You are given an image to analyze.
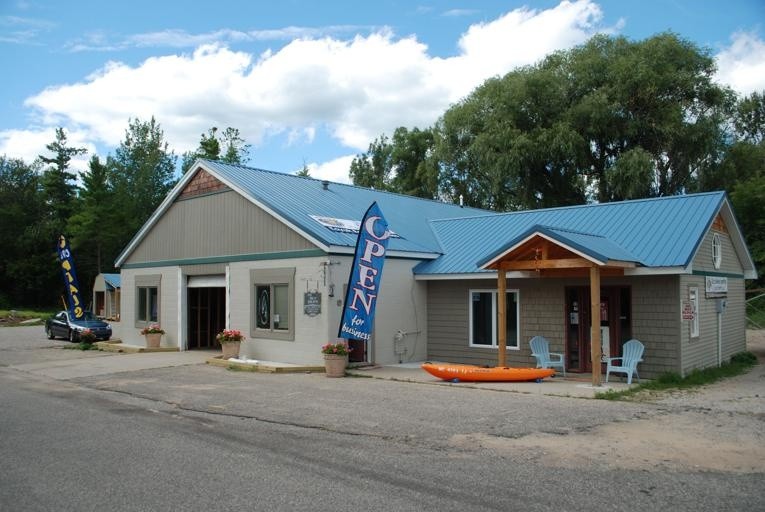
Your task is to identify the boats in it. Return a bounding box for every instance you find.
[419,360,558,384]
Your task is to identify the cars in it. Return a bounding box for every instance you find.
[44,310,113,343]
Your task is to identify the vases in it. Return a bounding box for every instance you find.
[145,333,161,349]
[82,338,93,345]
[324,353,347,378]
[221,341,240,359]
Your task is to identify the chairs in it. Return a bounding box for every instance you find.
[529,336,565,379]
[605,339,645,387]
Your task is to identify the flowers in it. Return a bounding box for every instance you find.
[79,328,96,339]
[321,337,352,355]
[216,329,245,343]
[140,323,166,335]
